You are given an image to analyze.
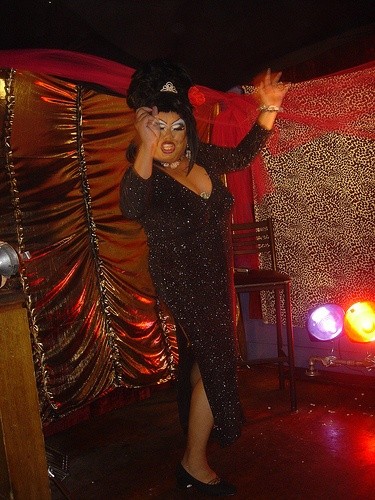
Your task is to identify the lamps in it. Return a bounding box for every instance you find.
[305,304,346,361]
[346,300,375,362]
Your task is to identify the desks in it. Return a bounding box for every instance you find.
[0,278,50,500]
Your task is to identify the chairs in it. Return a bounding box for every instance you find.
[231,219,295,411]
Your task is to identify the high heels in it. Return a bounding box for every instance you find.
[176,461,237,496]
